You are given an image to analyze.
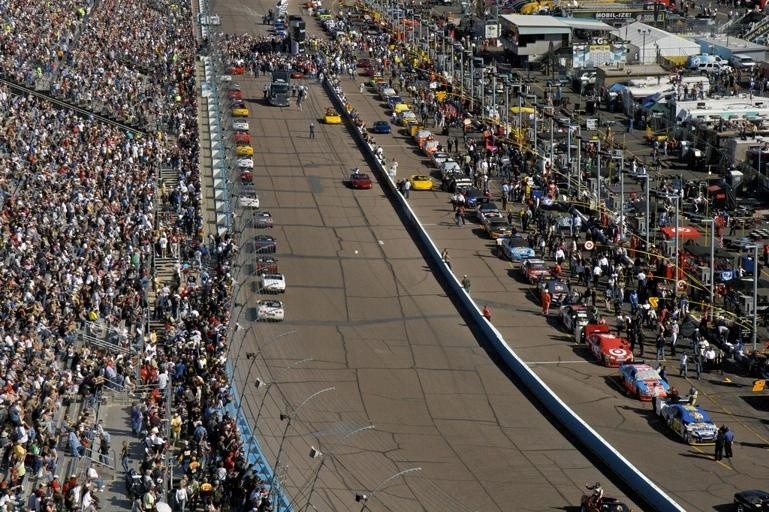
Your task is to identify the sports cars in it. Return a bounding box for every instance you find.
[581,495,633,511]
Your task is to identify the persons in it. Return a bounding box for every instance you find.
[0,1,768,510]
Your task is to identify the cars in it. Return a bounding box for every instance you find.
[219,66,289,322]
[733,490,769,511]
[267,19,288,40]
